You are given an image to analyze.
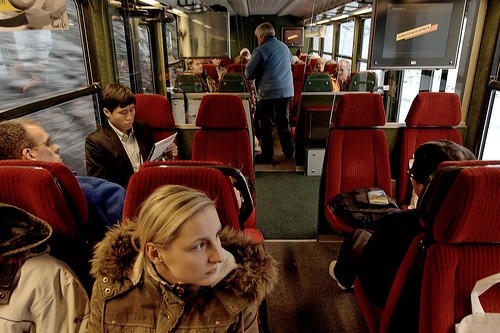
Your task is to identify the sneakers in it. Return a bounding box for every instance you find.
[329,259,355,291]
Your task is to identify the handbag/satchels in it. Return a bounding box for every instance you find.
[455,273,500,333]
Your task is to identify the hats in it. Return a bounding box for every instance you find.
[413,139,476,180]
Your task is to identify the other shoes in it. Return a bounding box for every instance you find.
[286,144,295,161]
[255,151,274,164]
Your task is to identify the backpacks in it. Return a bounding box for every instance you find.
[329,185,401,231]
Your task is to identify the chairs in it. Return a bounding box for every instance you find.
[122,160,256,251]
[0,159,94,289]
[130,94,179,161]
[351,161,499,331]
[323,94,394,234]
[418,165,499,333]
[399,92,463,228]
[191,93,256,206]
[172,53,381,140]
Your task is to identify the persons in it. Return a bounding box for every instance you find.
[245,22,295,163]
[290,47,358,92]
[0,120,126,238]
[329,140,479,309]
[315,26,325,37]
[85,83,178,187]
[212,47,259,104]
[186,56,220,94]
[0,200,91,333]
[84,184,282,333]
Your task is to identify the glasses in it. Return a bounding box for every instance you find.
[407,167,421,183]
[29,138,56,149]
